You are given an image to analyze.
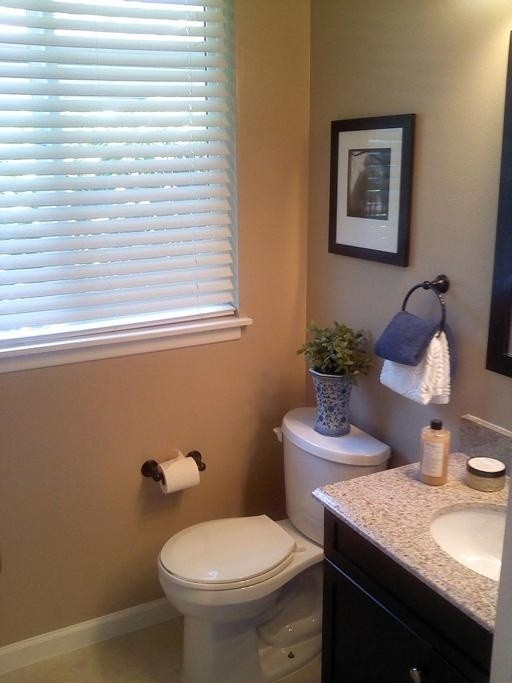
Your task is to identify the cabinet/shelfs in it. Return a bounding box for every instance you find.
[308,503,493,681]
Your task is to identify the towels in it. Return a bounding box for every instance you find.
[366,304,459,410]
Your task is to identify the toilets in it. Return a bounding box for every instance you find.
[156,404,392,683]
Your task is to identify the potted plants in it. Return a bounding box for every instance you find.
[296,317,376,439]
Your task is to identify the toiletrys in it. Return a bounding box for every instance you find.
[420,419,450,486]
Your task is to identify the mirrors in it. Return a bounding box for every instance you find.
[481,38,512,378]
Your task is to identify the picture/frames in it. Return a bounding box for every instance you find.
[327,110,420,269]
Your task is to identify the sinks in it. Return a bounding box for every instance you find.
[427,500,508,583]
